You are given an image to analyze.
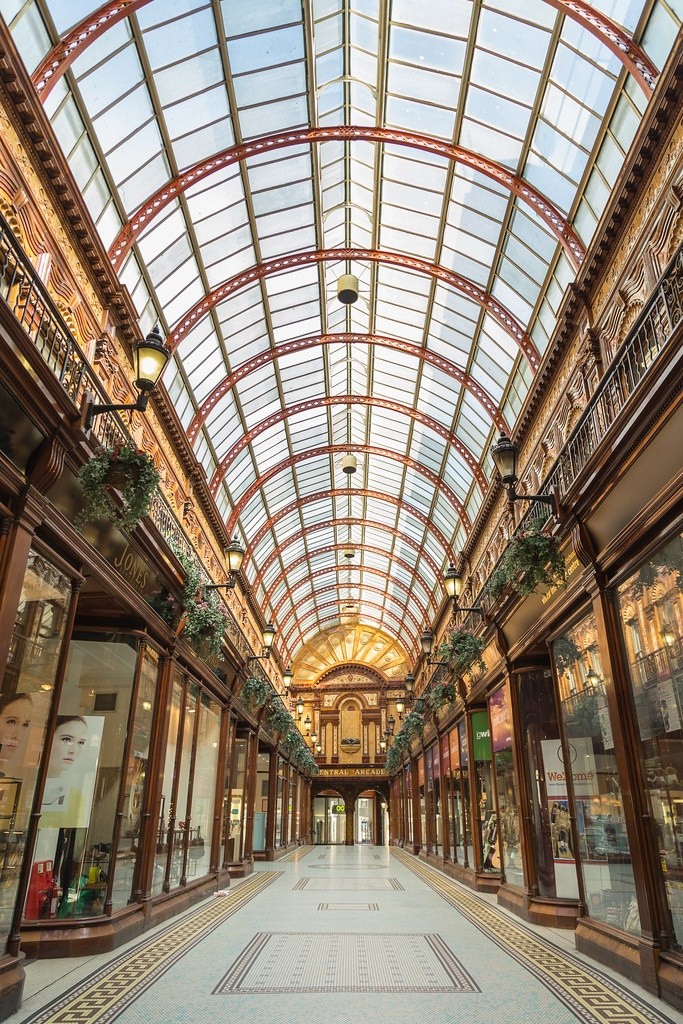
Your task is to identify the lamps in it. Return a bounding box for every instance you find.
[271,665,293,699]
[396,697,408,720]
[247,621,277,667]
[292,696,304,722]
[444,562,484,622]
[85,325,170,430]
[380,714,400,754]
[490,431,567,524]
[404,671,427,704]
[301,715,321,756]
[206,533,245,595]
[420,626,450,674]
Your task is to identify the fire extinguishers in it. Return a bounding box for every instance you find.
[39,879,61,919]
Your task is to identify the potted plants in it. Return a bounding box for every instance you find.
[340,738,361,754]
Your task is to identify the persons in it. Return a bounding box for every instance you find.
[559,838,568,854]
[0,694,35,829]
[38,715,94,828]
[600,827,627,851]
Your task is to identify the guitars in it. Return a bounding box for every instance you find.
[514,844,522,868]
[484,818,510,868]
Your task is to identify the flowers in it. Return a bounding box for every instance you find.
[430,681,456,706]
[185,596,230,663]
[76,442,158,531]
[435,625,487,675]
[487,518,569,597]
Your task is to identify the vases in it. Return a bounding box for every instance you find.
[201,624,217,637]
[108,464,136,492]
